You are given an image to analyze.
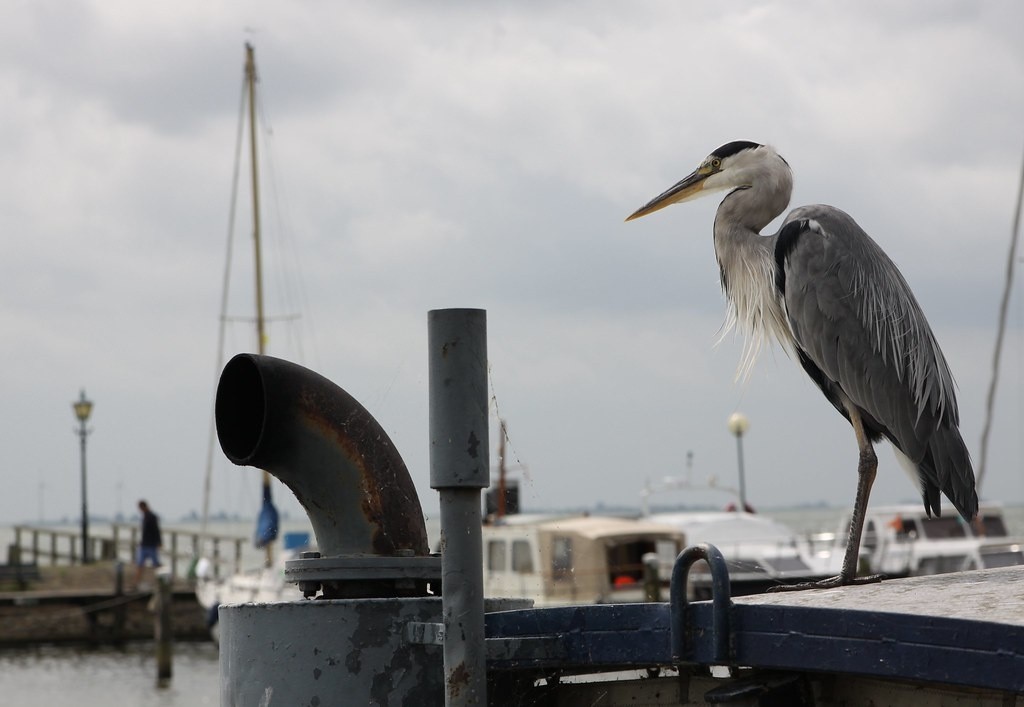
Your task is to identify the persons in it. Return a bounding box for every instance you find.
[138,501,162,566]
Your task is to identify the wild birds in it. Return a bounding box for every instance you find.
[623,138,982,591]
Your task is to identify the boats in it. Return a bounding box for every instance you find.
[192,39,1024,642]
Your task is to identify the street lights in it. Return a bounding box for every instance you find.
[65,388,96,564]
[728,413,746,513]
